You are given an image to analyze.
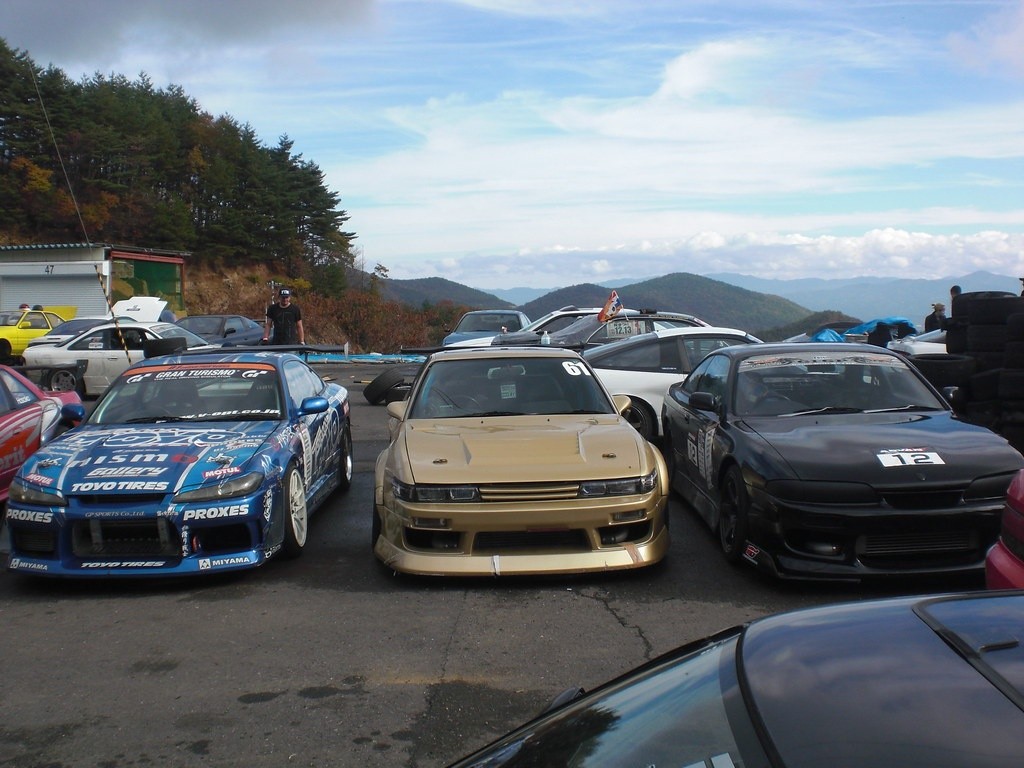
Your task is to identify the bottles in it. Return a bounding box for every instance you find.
[541,331,550,345]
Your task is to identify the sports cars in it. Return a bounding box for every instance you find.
[661,342,1024,592]
[578,327,878,441]
[4,343,353,579]
[0,364,83,507]
[373,342,671,577]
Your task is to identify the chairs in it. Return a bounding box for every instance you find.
[440,372,478,410]
[245,374,281,409]
[735,372,765,413]
[521,375,573,413]
[158,380,199,414]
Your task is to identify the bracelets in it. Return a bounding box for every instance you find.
[263,338,268,340]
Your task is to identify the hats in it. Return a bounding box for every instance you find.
[1019,277,1024,281]
[279,287,292,298]
[932,303,945,311]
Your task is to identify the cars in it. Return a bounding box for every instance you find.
[886,328,948,356]
[0,296,274,365]
[443,587,1024,768]
[442,304,712,354]
[20,321,245,402]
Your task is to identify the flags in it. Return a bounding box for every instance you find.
[598,290,623,322]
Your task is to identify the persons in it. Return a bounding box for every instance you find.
[924,303,946,332]
[260,288,305,346]
[19,303,29,308]
[898,322,916,339]
[33,305,43,311]
[869,322,892,347]
[950,285,961,298]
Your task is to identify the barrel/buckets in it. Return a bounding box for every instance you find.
[845,334,869,344]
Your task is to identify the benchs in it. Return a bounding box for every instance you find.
[200,394,247,411]
